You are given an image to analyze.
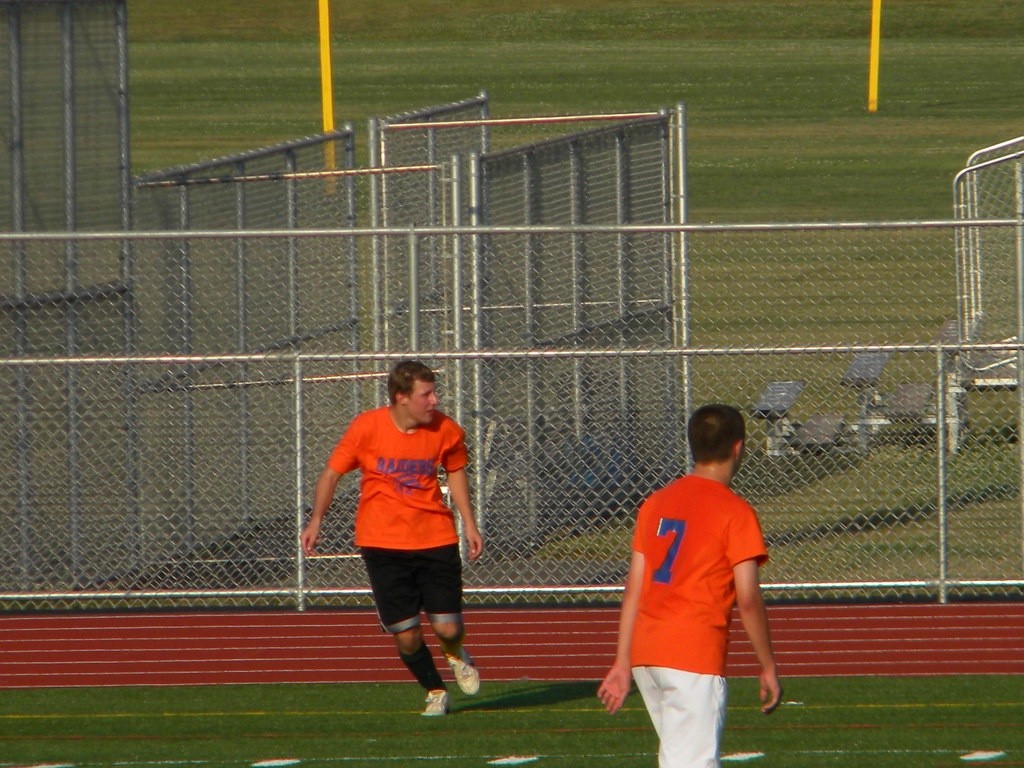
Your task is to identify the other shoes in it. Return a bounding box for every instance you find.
[440,644,480,695]
[421,690,448,717]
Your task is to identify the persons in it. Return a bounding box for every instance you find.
[299,357,484,715]
[598,403,783,768]
